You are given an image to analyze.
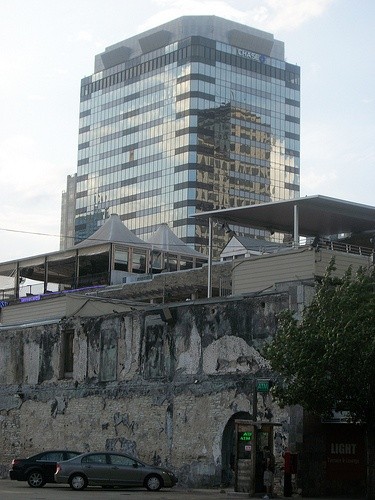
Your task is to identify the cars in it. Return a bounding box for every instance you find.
[8,450,83,488]
[54,451,177,492]
